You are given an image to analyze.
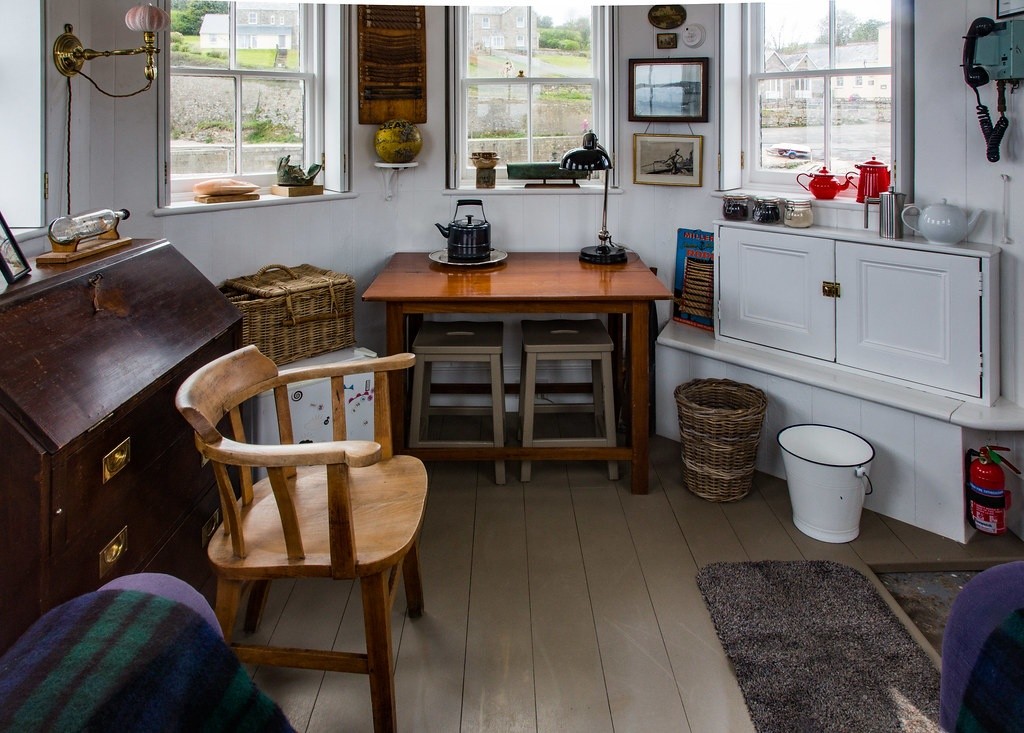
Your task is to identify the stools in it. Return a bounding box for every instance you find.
[408,320,507,485]
[521,318,621,482]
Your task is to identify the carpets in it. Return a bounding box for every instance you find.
[695,561,942,733]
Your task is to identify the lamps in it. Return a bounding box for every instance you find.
[559,133,628,265]
[54,1,171,101]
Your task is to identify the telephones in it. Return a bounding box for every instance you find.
[960,17,1024,87]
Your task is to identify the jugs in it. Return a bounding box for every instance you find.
[864,186,907,239]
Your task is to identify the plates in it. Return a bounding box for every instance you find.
[428,248,507,265]
[193,185,260,195]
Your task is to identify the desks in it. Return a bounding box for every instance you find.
[360,253,675,496]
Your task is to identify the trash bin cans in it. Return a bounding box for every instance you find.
[675,379,767,505]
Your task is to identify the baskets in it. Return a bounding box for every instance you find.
[673,378,766,502]
[215,263,355,366]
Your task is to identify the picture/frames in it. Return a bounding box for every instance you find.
[628,56,709,124]
[632,133,703,187]
[0,213,31,283]
[657,34,677,50]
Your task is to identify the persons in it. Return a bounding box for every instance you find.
[939,560,1024,733]
[0,572,297,733]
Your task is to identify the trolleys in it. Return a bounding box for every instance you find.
[766,143,811,159]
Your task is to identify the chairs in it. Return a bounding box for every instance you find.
[176,344,429,731]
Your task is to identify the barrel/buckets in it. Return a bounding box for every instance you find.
[777,424,875,543]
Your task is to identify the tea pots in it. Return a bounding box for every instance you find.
[901,198,986,246]
[846,157,892,203]
[435,199,495,263]
[796,167,853,199]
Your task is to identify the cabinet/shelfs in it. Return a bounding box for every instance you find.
[712,219,1002,407]
[1,238,243,657]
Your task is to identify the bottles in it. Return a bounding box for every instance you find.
[49,207,130,245]
[784,199,813,228]
[723,194,753,221]
[753,196,786,225]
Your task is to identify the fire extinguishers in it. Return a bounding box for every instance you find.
[968,445,1021,535]
